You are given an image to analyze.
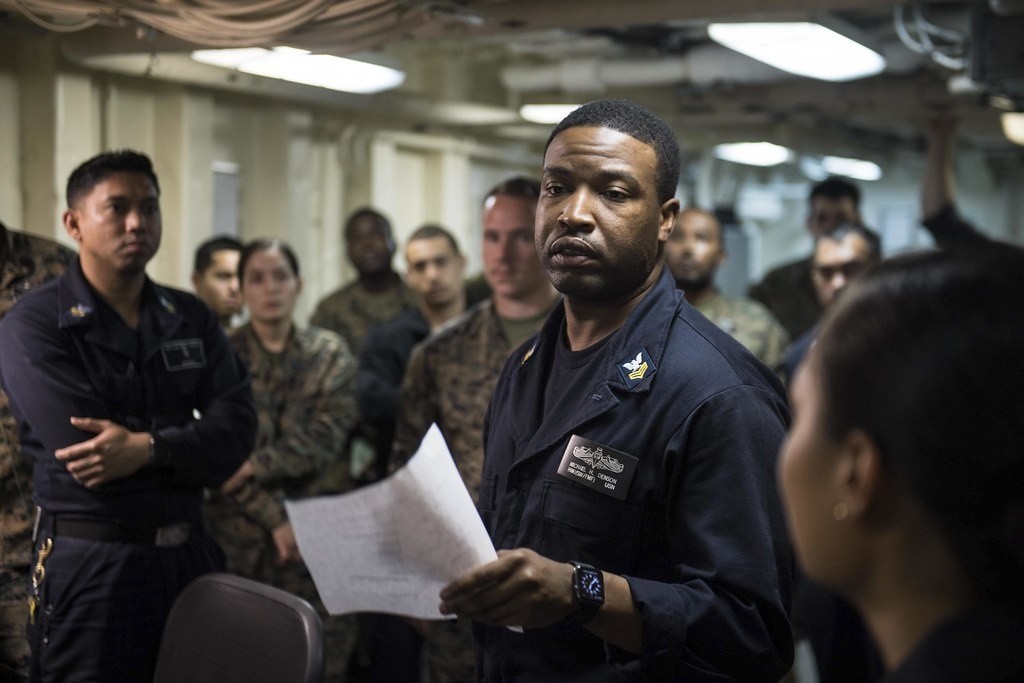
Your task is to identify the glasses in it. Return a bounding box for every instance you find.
[809,260,868,280]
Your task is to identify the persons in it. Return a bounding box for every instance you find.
[920,70,1024,277]
[386,177,569,683]
[750,175,886,375]
[775,240,1024,682]
[0,148,258,683]
[441,104,813,682]
[662,206,793,369]
[204,242,361,682]
[310,206,416,353]
[192,233,246,337]
[317,227,488,683]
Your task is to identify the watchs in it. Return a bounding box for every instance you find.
[557,561,606,628]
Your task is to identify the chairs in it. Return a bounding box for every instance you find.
[156,573,327,682]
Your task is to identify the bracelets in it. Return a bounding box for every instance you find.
[150,438,159,472]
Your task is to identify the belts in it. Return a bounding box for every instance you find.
[39,510,202,549]
